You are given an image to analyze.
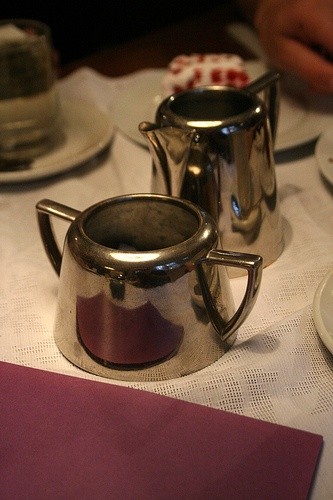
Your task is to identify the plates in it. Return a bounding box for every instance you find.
[0,93,114,181]
[310,264,332,362]
[112,63,327,153]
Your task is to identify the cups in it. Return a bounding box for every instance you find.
[138,70,286,276]
[0,19,66,162]
[35,192,263,382]
